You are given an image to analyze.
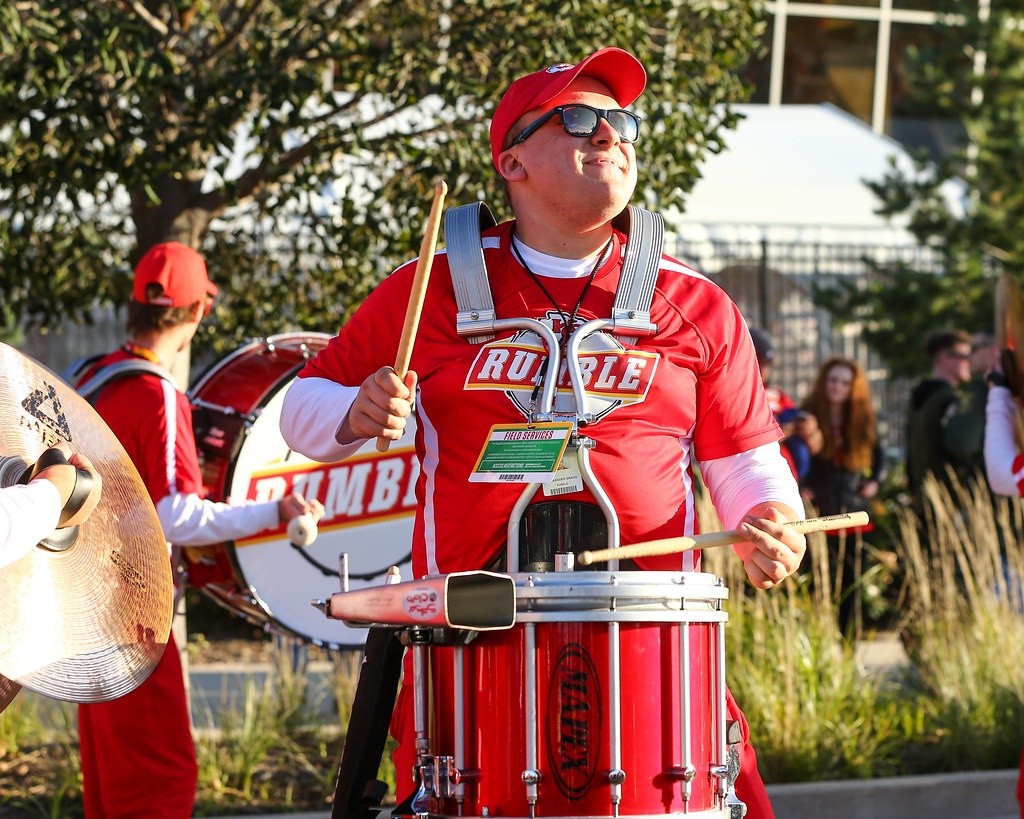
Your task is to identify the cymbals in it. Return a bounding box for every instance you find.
[0,339,177,708]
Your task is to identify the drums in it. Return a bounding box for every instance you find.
[185,327,422,651]
[395,566,735,819]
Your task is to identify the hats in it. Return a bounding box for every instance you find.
[133,243,218,306]
[489,48,647,176]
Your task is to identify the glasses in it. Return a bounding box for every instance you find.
[504,104,641,149]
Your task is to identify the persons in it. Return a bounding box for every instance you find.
[749,328,1023,633]
[280,43,804,819]
[0,243,306,819]
